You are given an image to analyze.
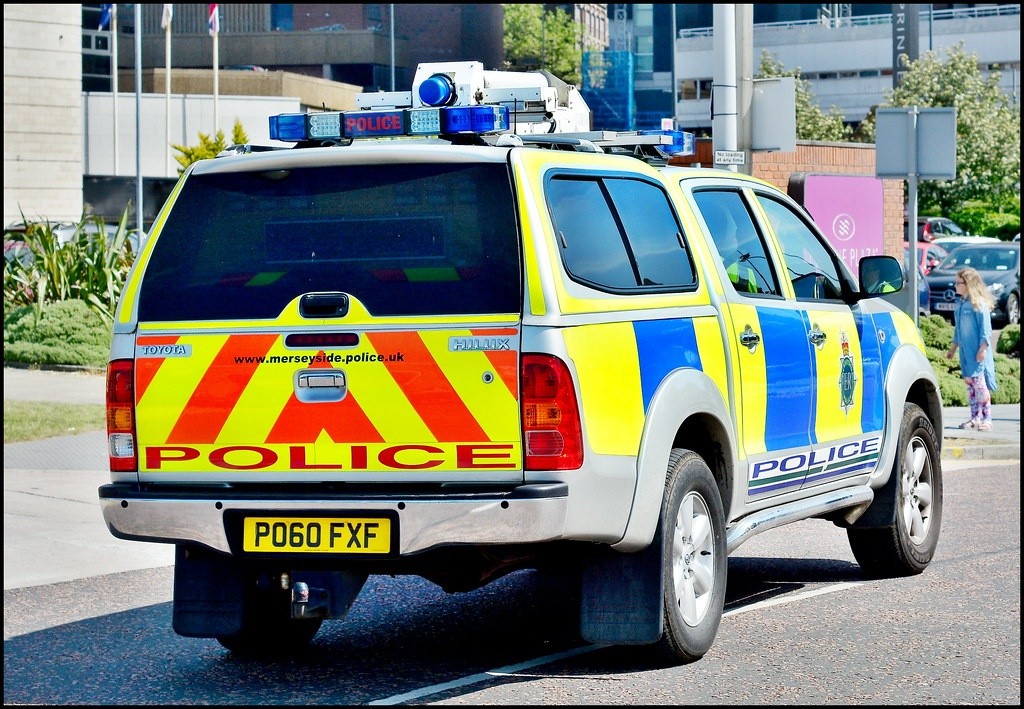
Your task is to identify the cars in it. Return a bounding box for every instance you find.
[4,219,144,301]
[903,216,1020,330]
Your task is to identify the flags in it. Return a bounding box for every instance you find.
[160,4,173,28]
[207,4,220,38]
[97,4,116,33]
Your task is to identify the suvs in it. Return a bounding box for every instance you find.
[98,59,944,663]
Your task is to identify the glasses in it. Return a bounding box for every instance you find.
[956,281,966,285]
[863,268,877,273]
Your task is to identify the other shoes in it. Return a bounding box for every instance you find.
[959,419,977,430]
[977,422,993,431]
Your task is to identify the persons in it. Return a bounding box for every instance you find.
[946,266,999,432]
